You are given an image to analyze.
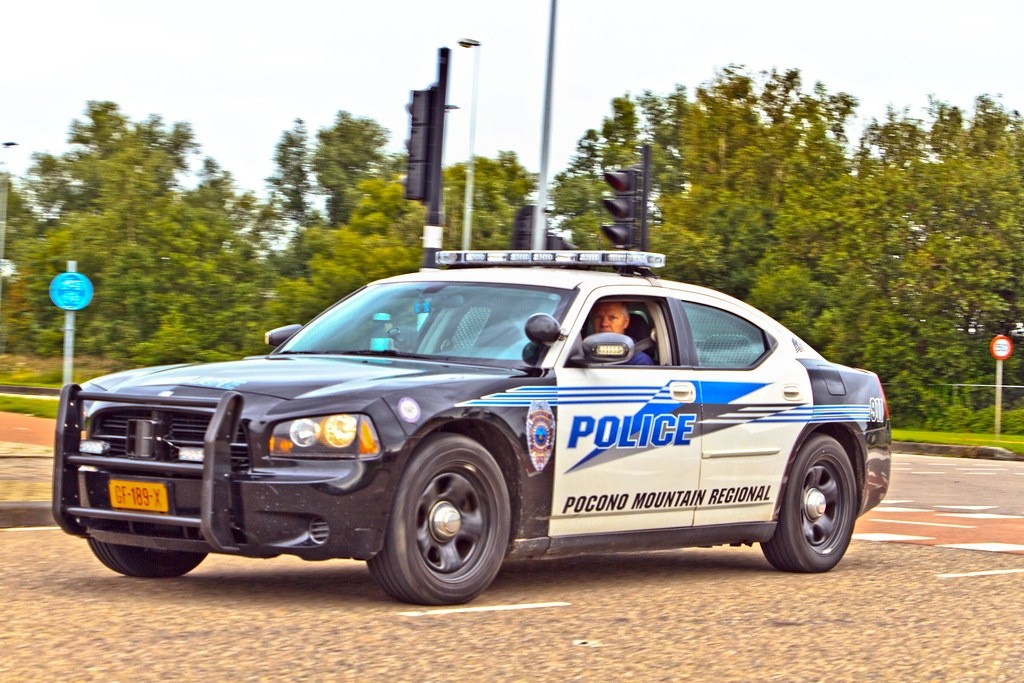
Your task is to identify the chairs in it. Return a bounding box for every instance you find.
[624,313,655,358]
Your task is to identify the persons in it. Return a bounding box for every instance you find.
[593,301,654,365]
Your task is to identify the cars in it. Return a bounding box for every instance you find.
[53,250,892,606]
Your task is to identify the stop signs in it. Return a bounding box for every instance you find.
[990,335,1013,359]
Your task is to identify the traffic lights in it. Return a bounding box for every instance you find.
[599,171,636,247]
[405,88,430,202]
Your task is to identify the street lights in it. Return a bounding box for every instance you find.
[458,37,481,249]
